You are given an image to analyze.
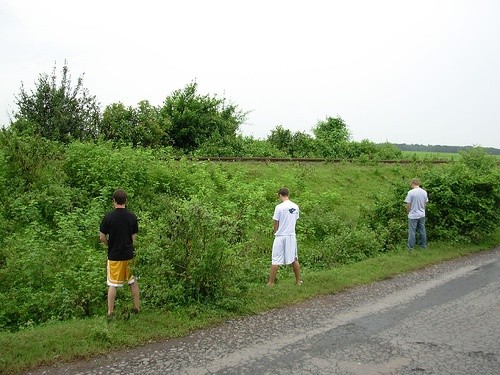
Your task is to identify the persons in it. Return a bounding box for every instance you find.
[404,178,429,249]
[99,189,141,317]
[264,188,304,287]
